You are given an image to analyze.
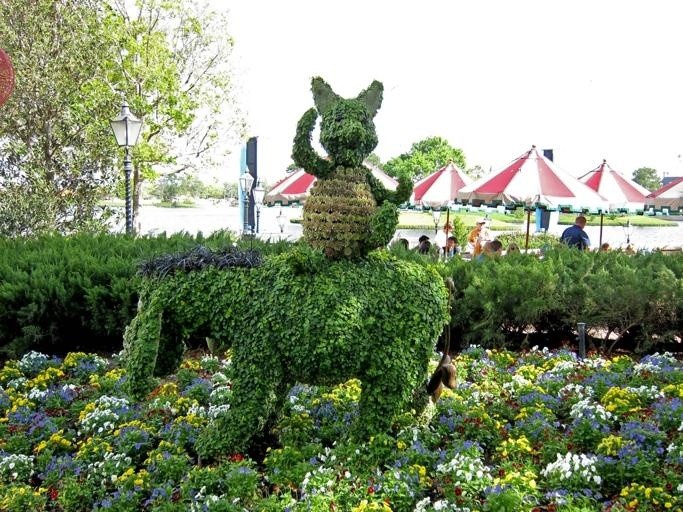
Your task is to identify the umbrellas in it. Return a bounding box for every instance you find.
[409,157,474,262]
[265,156,399,202]
[645,176,683,199]
[456,144,609,254]
[577,158,651,246]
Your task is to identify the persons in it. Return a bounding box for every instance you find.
[561,216,590,249]
[399,220,520,260]
[483,213,492,241]
[602,243,610,252]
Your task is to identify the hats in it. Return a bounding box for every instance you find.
[476,220,487,225]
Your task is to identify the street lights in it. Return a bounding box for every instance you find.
[621,219,635,249]
[276,209,287,233]
[106,97,146,235]
[239,166,252,236]
[252,179,264,233]
[430,208,442,234]
[482,214,492,232]
